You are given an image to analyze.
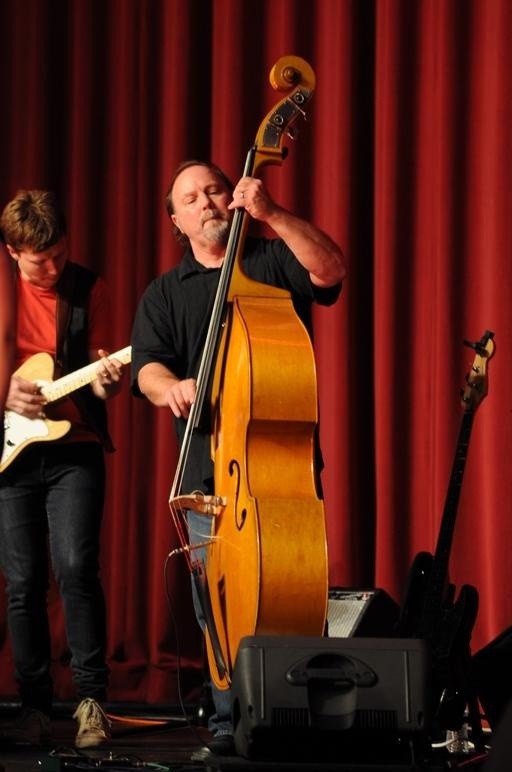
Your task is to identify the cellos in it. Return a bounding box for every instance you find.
[168,54,331,695]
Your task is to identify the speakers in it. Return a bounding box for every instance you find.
[326,587,402,638]
[232,635,426,753]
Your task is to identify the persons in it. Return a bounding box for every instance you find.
[1,189,124,750]
[127,159,350,763]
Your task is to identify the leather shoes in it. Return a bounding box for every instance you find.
[201,735,234,755]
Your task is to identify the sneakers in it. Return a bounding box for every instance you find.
[2,709,50,746]
[72,698,111,748]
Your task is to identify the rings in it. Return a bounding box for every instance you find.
[240,191,245,198]
[103,373,110,378]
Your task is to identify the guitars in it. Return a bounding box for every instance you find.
[0,345,133,474]
[409,330,496,665]
